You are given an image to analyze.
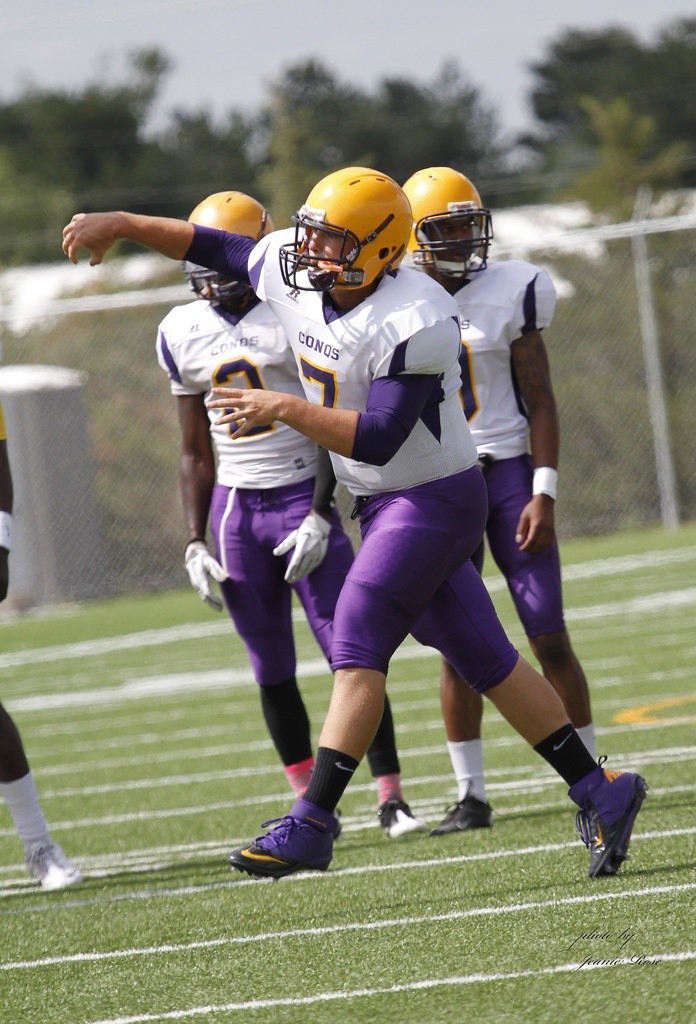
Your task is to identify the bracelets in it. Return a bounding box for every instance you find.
[185,538,207,553]
[532,467,558,501]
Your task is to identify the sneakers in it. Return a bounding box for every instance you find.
[568,755,648,878]
[429,781,493,835]
[227,799,339,880]
[27,844,81,891]
[378,797,424,839]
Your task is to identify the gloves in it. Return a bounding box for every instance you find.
[271,510,332,584]
[184,538,228,613]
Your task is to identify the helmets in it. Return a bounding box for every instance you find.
[278,166,414,292]
[401,166,495,279]
[181,191,274,308]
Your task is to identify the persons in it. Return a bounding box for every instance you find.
[63,166,649,879]
[1,392,81,892]
